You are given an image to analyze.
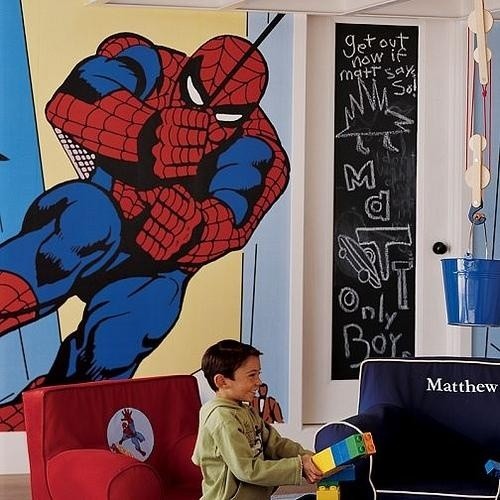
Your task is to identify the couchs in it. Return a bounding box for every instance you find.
[313,357,500,500]
[22,375,203,500]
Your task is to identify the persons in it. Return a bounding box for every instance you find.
[248,382,284,424]
[0,32,349,432]
[190,341,321,500]
